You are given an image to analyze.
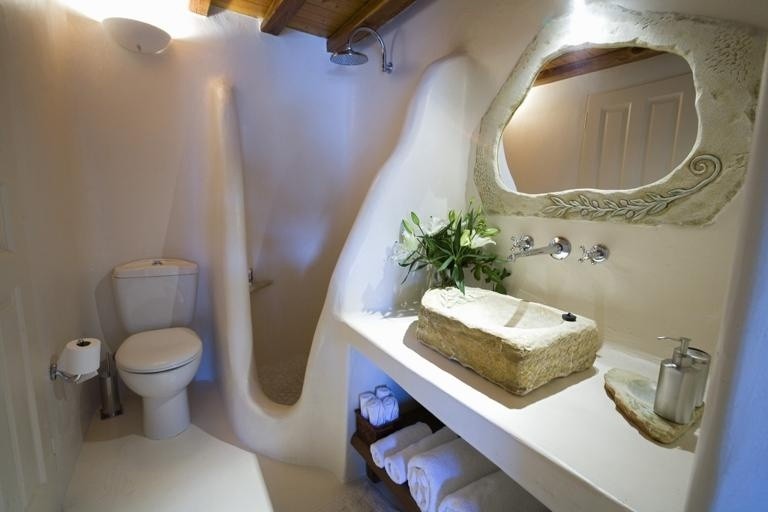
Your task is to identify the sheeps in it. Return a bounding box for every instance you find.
[509,237,571,261]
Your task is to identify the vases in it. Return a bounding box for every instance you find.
[392,199,511,295]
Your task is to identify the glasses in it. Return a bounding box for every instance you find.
[100,18,172,52]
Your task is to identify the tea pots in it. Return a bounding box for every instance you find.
[472,0,768,227]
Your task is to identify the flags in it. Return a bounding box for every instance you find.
[426,263,449,287]
